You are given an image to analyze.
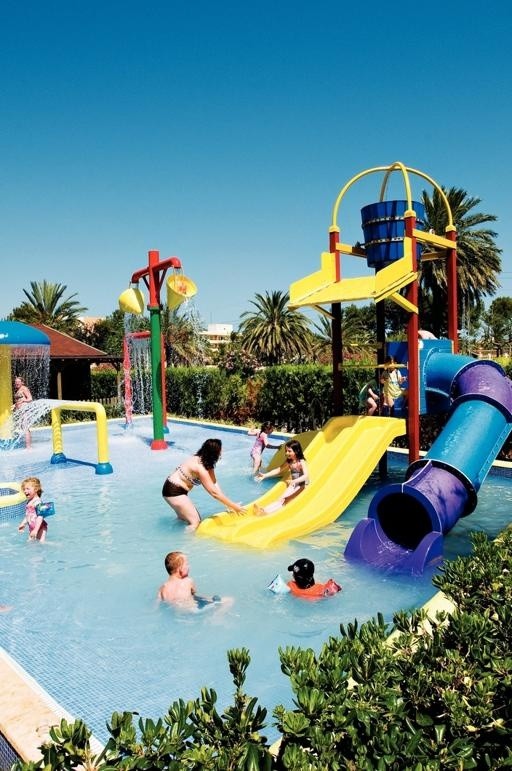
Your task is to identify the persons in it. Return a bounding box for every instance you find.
[162,438,249,534]
[157,551,234,625]
[251,420,284,478]
[18,477,47,545]
[380,357,407,416]
[253,440,309,515]
[286,558,342,601]
[358,370,388,415]
[13,376,34,449]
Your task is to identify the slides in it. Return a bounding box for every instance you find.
[345,338,512,576]
[196,416,407,548]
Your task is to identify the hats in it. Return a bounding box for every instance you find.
[288,559,315,577]
